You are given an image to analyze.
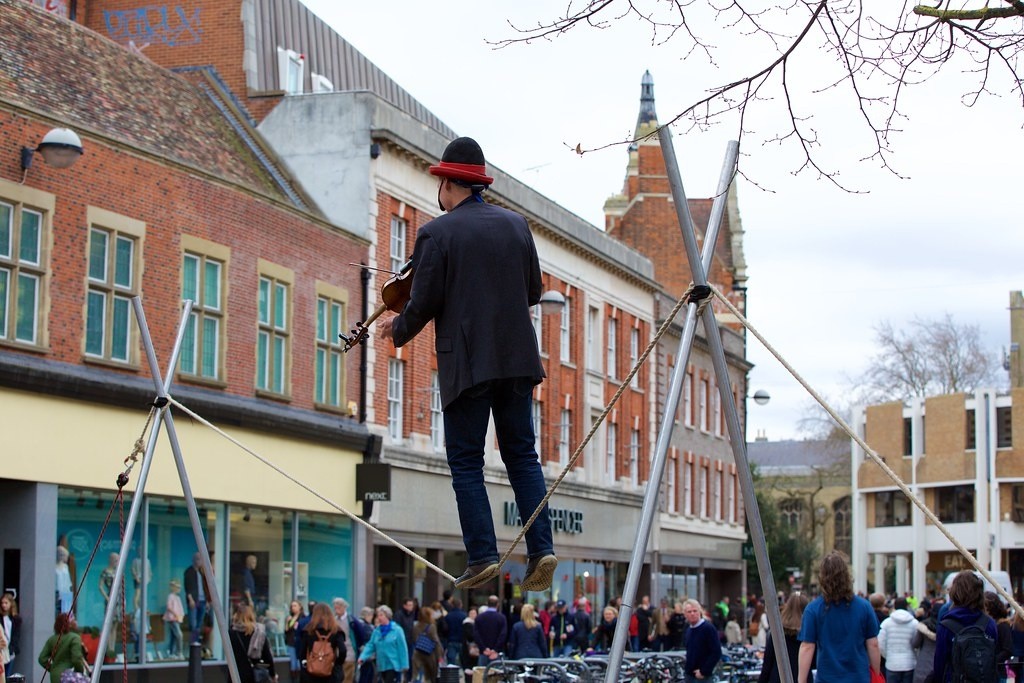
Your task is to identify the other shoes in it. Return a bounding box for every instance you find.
[455,559,499,589]
[519,554,557,592]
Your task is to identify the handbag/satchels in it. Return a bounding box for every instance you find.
[60,668,91,683]
[251,662,272,683]
[414,635,436,656]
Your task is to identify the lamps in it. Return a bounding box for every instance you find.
[22,126,83,169]
[94,490,104,509]
[263,509,272,523]
[75,488,85,507]
[539,290,566,315]
[745,390,770,404]
[242,506,250,522]
[165,498,173,514]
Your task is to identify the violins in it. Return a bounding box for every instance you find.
[338,264,414,353]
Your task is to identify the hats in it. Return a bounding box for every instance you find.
[556,600,566,608]
[428,137,493,186]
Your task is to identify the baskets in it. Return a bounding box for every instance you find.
[472,667,499,683]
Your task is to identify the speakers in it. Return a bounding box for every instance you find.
[355,463,392,502]
[370,143,386,156]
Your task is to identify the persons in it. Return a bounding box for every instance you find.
[0,552,1024,683]
[56,546,258,663]
[379,137,555,590]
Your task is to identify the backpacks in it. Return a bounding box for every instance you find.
[750,620,760,636]
[306,629,335,675]
[939,612,1000,683]
